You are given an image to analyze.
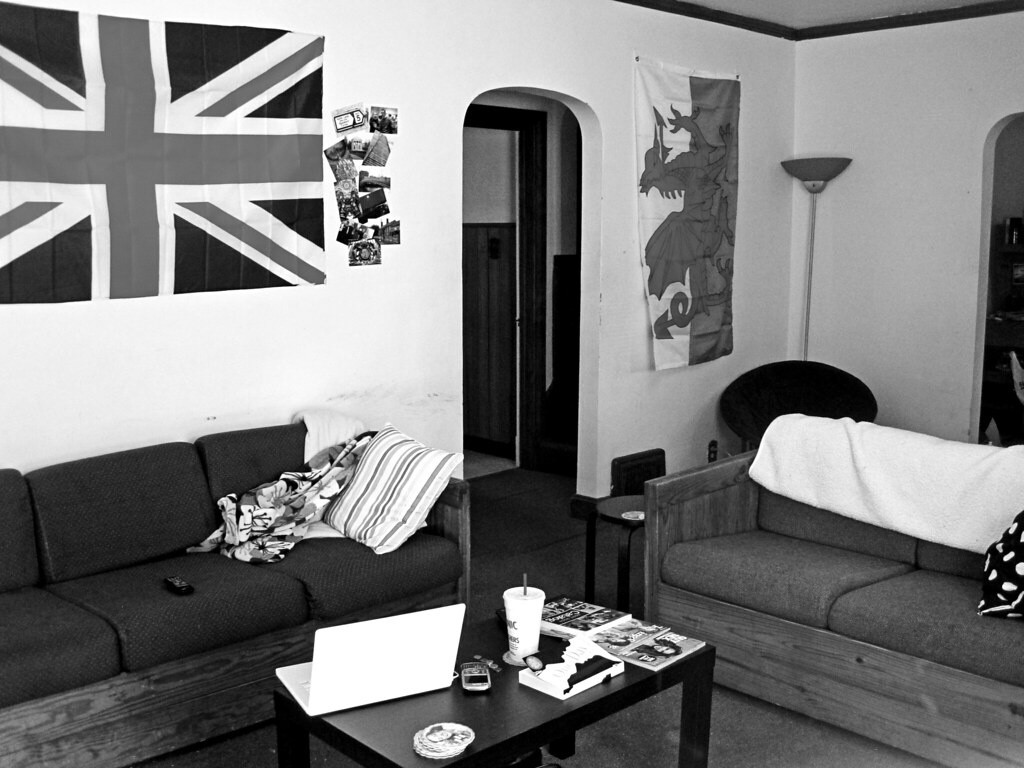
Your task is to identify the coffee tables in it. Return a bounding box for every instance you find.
[272,594,717,768]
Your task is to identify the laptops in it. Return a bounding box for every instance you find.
[274,603,466,717]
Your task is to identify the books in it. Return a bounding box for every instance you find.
[517,634,625,702]
[541,594,706,672]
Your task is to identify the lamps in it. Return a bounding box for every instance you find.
[780,157,853,359]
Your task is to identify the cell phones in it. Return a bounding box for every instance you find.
[461,662,491,691]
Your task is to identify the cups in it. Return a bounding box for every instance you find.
[502,586,546,662]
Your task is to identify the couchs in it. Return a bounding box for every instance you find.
[643,413,1024,768]
[0,422,471,768]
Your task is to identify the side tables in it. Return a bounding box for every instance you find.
[585,494,643,613]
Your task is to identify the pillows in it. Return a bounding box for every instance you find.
[976,509,1024,620]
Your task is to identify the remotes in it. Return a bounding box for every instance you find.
[165,575,194,595]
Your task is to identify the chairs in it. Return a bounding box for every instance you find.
[721,360,879,453]
